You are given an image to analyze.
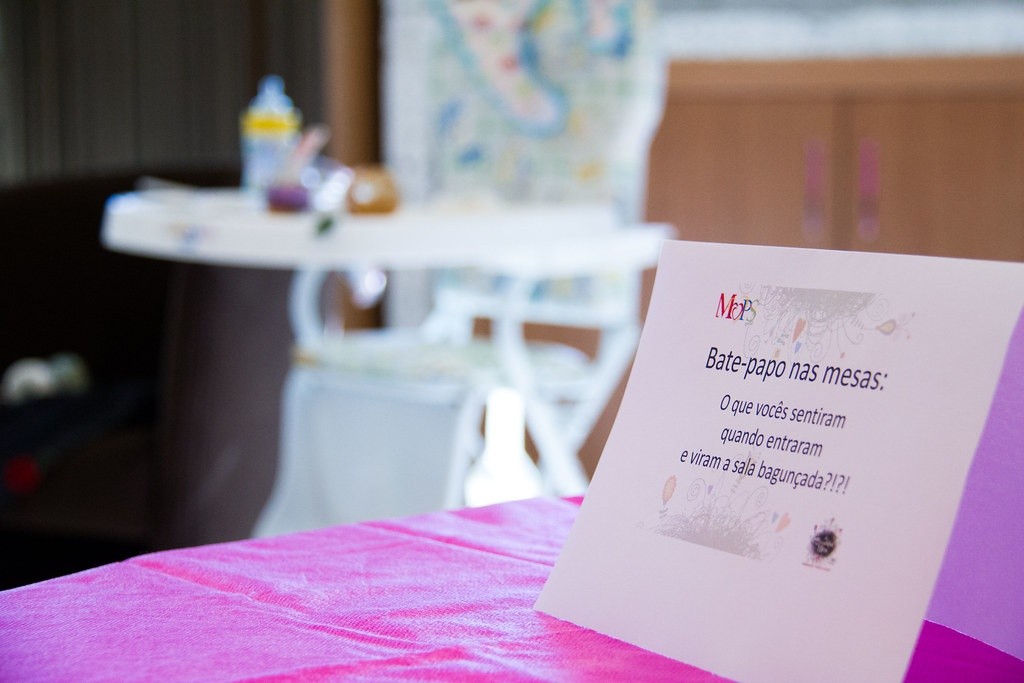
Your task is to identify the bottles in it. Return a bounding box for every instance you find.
[239,75,307,209]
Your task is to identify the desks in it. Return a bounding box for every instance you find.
[101,193,681,538]
[0,494,1024,683]
